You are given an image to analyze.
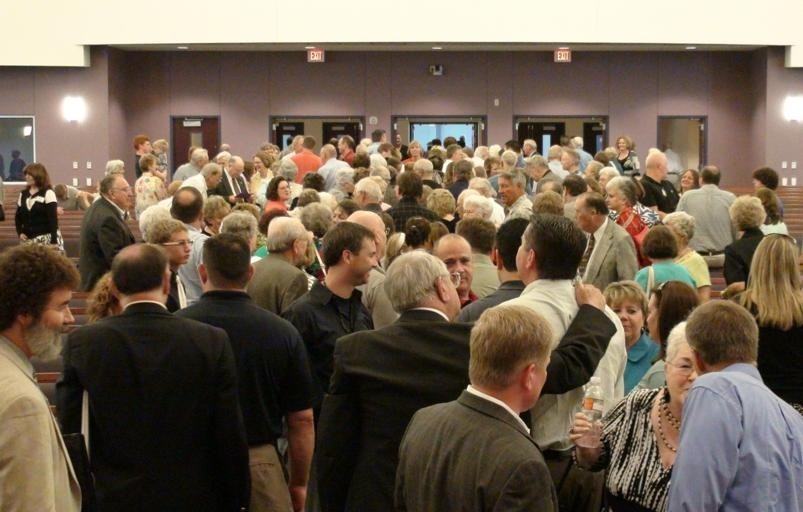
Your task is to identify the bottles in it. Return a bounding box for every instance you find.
[576,377,604,449]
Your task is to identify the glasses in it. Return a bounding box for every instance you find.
[449,273,461,287]
[161,240,193,246]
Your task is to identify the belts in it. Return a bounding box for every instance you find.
[698,251,724,255]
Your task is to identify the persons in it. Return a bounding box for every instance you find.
[55,242,252,512]
[1,244,84,510]
[394,306,554,511]
[666,298,803,510]
[5,149,26,180]
[57,130,802,511]
[14,164,65,252]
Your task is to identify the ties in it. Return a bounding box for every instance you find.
[580,234,595,277]
[175,275,187,310]
[233,178,240,195]
